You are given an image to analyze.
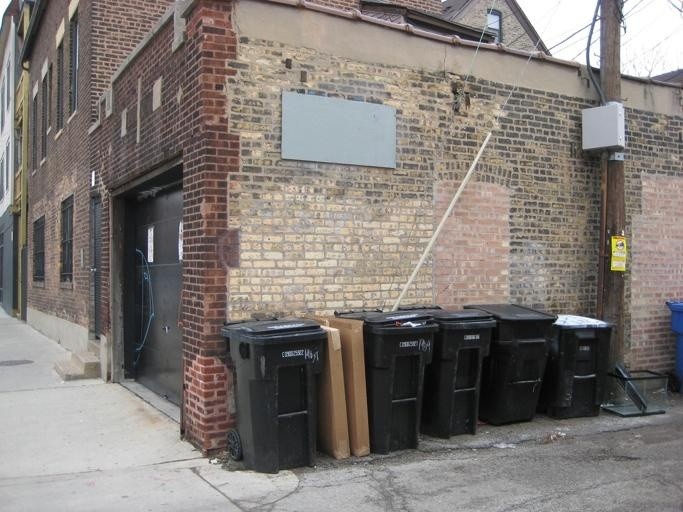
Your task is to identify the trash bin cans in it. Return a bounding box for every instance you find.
[335,308,440,457]
[399,305,497,439]
[665,301,683,394]
[220,317,330,475]
[464,304,558,425]
[536,316,613,418]
